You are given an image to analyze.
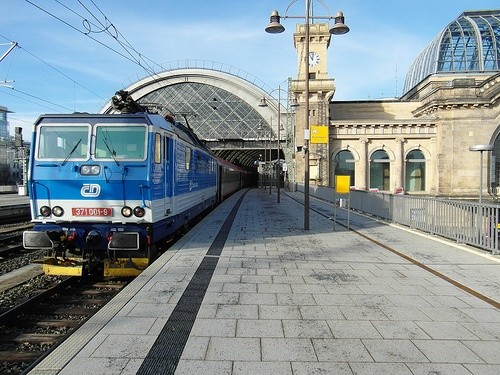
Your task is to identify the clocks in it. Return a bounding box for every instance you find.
[304,51,320,67]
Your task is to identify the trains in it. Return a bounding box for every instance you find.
[23,90,248,277]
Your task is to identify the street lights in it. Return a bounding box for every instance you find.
[259,84,300,205]
[258,122,284,196]
[265,0,350,230]
